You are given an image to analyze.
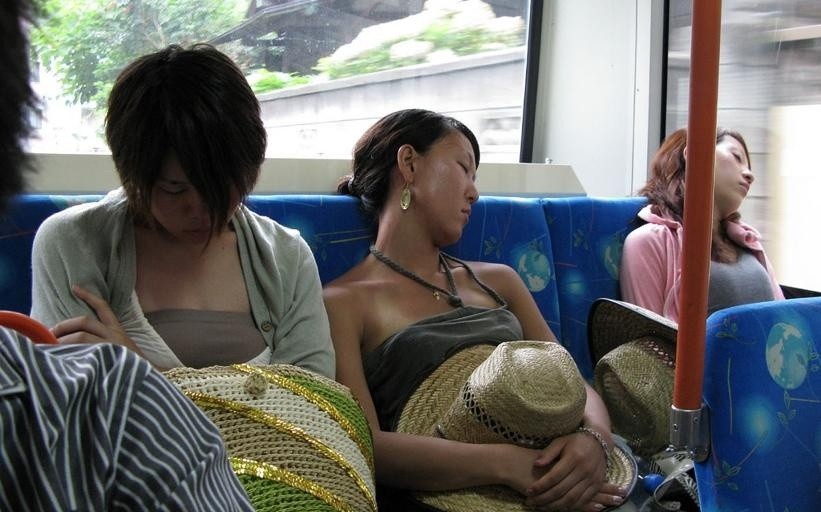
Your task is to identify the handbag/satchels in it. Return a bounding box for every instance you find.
[160,362,379,511]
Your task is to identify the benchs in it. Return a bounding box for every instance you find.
[1,187,651,512]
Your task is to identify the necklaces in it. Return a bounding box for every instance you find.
[392,255,443,303]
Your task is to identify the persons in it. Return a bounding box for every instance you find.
[1,1,257,512]
[322,106,637,509]
[619,124,789,511]
[29,41,337,380]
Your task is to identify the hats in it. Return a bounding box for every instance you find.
[393,336,642,512]
[584,294,678,463]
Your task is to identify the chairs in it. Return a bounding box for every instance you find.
[693,297,820,512]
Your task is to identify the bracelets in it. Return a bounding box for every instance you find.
[576,425,614,479]
